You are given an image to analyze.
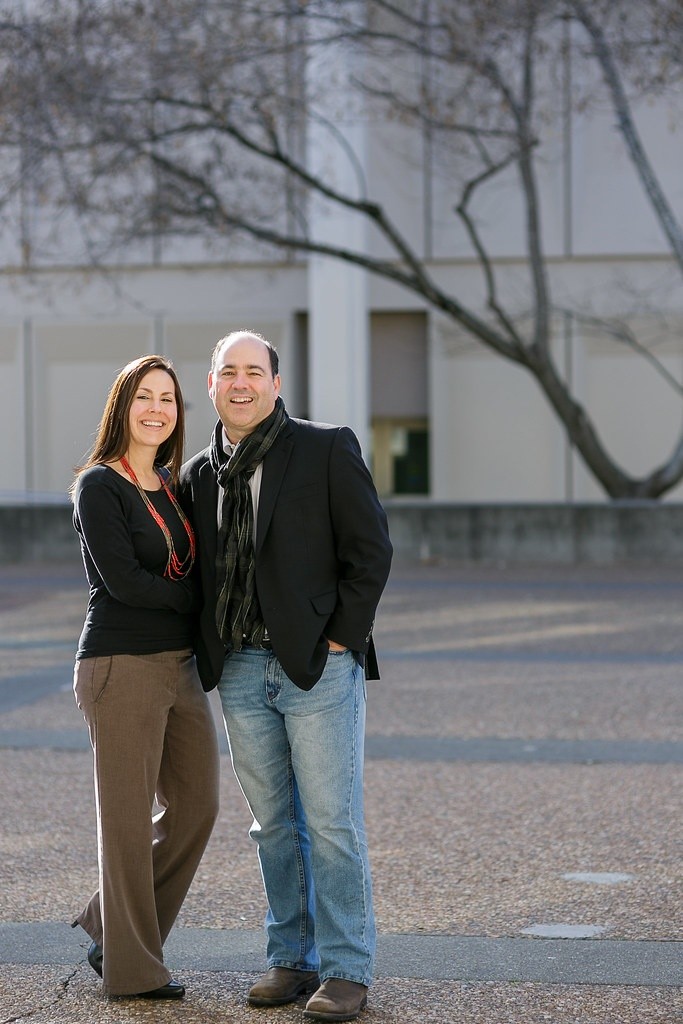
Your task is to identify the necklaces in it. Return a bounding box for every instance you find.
[120,453,197,580]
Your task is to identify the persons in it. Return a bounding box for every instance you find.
[180,329,392,1021]
[71,355,220,993]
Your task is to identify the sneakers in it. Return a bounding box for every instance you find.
[302,977,368,1021]
[246,966,320,1006]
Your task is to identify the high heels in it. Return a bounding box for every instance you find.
[71,919,103,978]
[134,979,185,999]
